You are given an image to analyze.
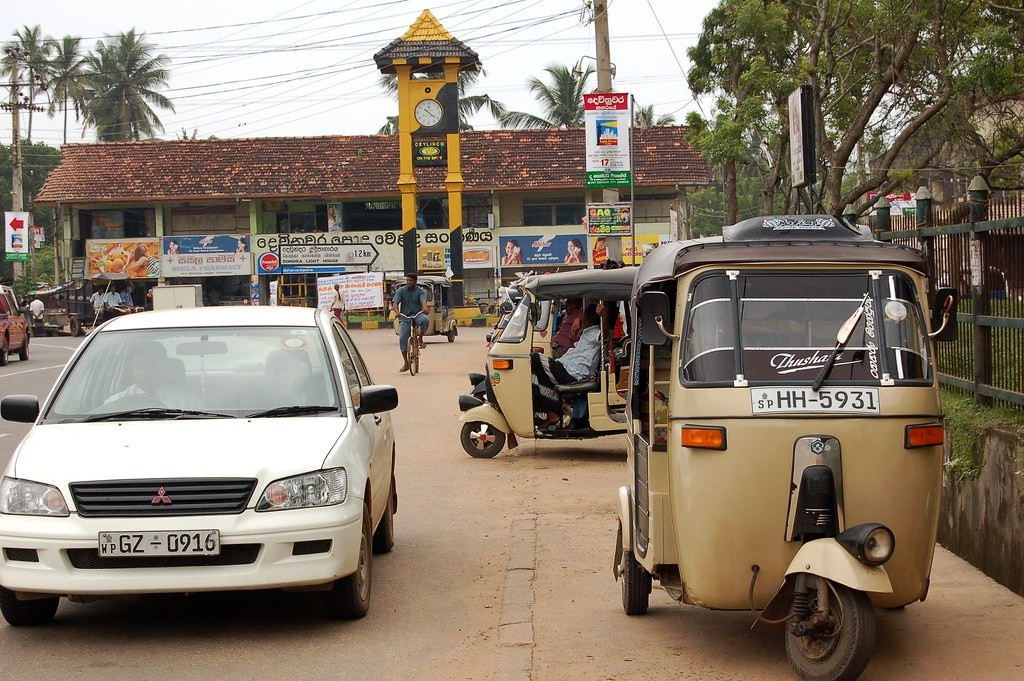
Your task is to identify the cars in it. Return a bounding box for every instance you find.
[1,306,399,622]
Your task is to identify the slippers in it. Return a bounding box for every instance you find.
[400,365,409,372]
[419,341,426,349]
[540,416,559,429]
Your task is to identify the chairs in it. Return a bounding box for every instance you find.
[161,358,186,393]
[240,351,319,407]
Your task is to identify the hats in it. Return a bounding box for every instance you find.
[128,341,167,369]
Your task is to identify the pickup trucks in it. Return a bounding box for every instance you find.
[0,284,31,366]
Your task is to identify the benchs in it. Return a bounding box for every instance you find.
[554,375,599,429]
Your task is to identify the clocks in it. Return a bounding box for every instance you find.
[416,100,443,126]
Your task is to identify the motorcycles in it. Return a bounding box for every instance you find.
[458,265,642,459]
[389,274,458,343]
[613,213,960,681]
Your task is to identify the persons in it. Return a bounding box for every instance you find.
[21,281,135,336]
[502,240,522,264]
[127,244,160,275]
[564,238,585,263]
[392,273,429,372]
[330,284,345,318]
[101,341,204,411]
[531,299,622,428]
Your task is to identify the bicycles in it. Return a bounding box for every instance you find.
[393,311,426,376]
[464,295,489,315]
